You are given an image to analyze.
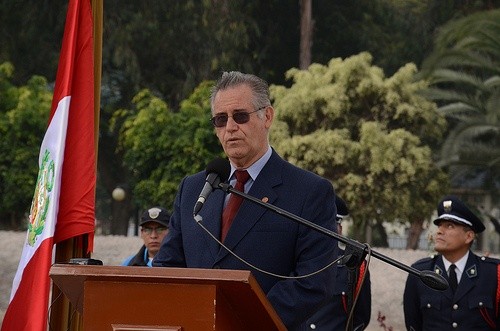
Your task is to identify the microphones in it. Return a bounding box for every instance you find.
[193,157,231,215]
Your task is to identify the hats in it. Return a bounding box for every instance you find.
[334,195,350,223]
[432,197,486,234]
[138,206,170,227]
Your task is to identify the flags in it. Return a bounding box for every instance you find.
[0,1,95,331]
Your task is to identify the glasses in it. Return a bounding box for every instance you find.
[209,106,267,128]
[142,226,167,234]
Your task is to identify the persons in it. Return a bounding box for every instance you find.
[335,195,372,331]
[149,71,340,331]
[121,207,171,268]
[403,196,500,331]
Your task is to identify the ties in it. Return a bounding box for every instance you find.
[218,169,250,244]
[449,264,458,295]
